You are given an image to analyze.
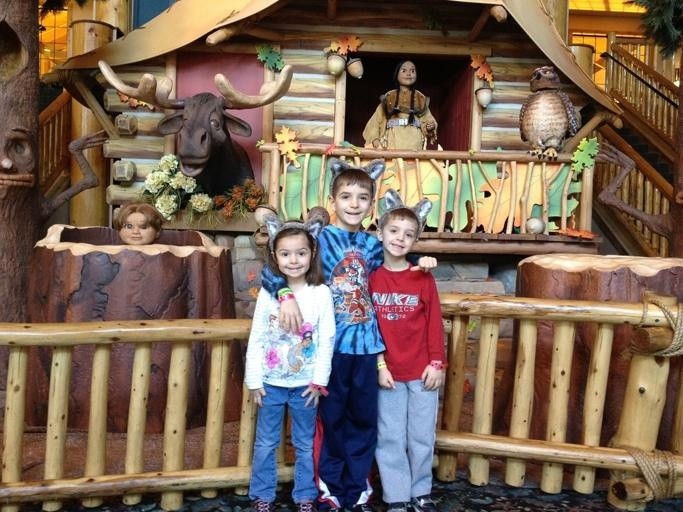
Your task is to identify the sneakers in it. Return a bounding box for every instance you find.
[318,496,435,512]
[249,499,271,512]
[295,500,317,512]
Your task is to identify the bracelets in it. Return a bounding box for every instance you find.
[428,360,449,370]
[276,288,291,297]
[277,293,296,302]
[375,361,388,369]
[307,382,330,397]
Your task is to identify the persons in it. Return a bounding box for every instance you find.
[375,187,447,511]
[359,58,442,152]
[112,203,166,246]
[254,159,438,512]
[241,215,337,512]
